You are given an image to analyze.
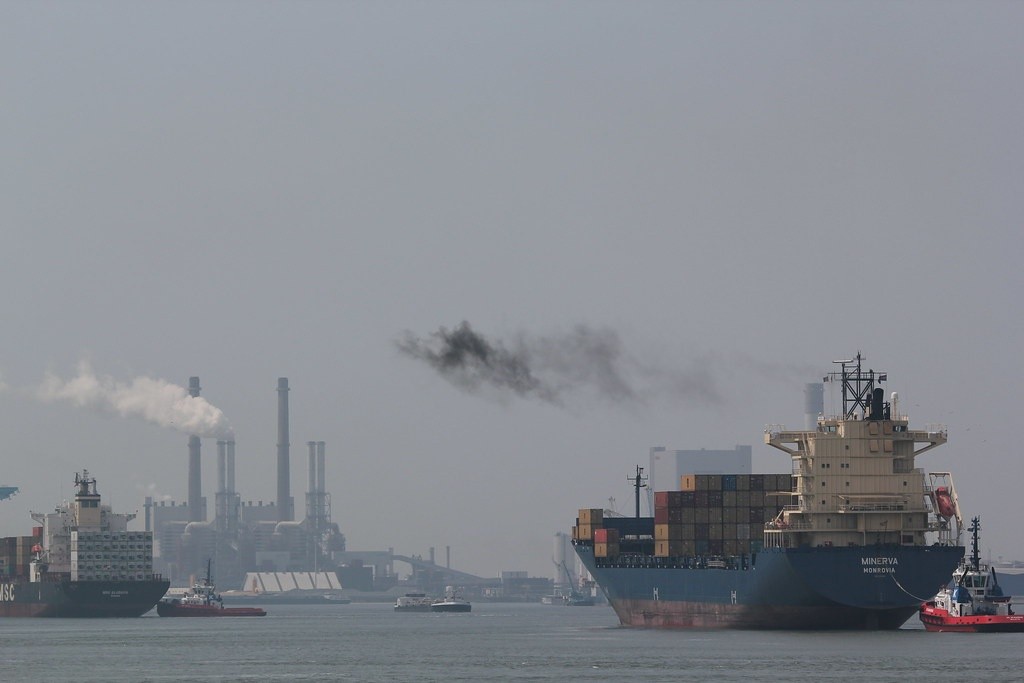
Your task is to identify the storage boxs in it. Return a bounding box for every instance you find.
[571,474,801,556]
[0,527,153,581]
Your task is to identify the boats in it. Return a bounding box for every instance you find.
[936,486,954,524]
[541,588,595,606]
[156,558,267,617]
[394,593,431,612]
[917,515,1024,632]
[570,348,968,630]
[431,585,471,612]
[0,469,171,619]
